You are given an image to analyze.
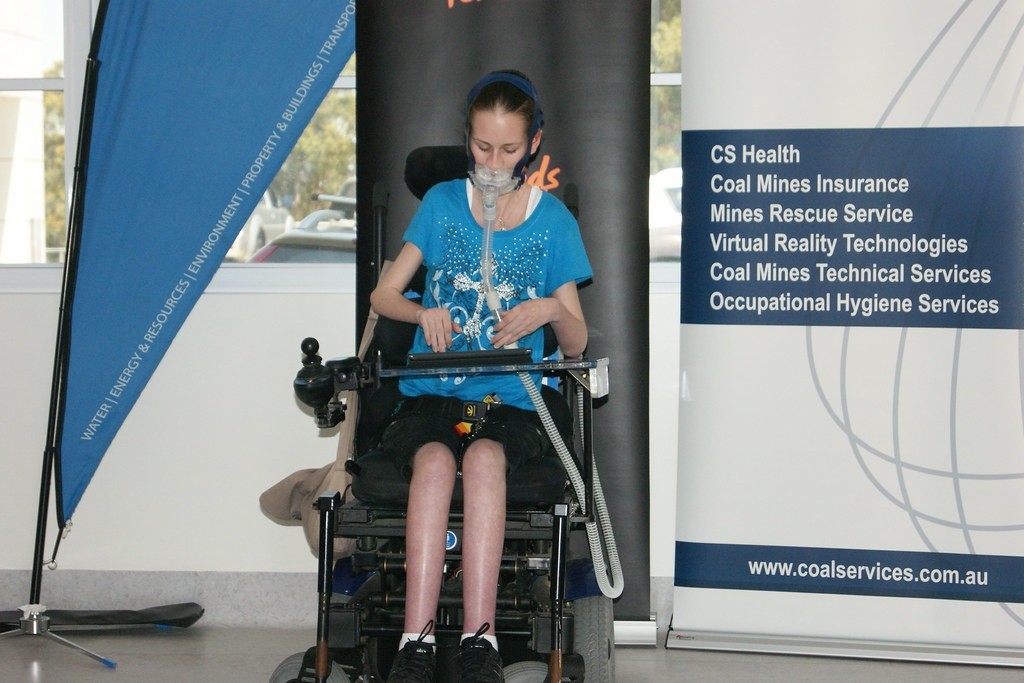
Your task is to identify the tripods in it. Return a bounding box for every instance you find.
[0,0,171,668]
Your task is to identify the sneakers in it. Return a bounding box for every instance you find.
[452,622,505,683]
[386,620,441,683]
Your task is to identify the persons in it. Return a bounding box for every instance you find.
[370,69,593,683]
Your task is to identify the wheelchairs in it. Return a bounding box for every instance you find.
[295,143,615,683]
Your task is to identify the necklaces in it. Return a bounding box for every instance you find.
[498,218,507,231]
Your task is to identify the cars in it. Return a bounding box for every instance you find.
[222,189,297,259]
[242,209,357,262]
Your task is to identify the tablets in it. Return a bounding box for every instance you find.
[406,349,532,367]
